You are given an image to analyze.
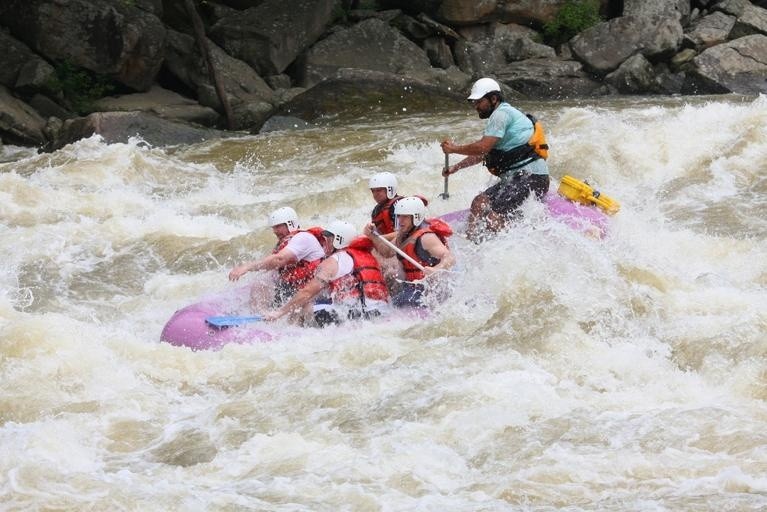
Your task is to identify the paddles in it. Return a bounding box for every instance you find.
[205,310,291,328]
[439,140,451,202]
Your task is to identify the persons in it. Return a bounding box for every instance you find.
[226,170,457,331]
[441,76,552,243]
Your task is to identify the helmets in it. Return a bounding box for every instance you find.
[268,207,299,233]
[368,172,399,200]
[325,221,354,250]
[393,196,425,226]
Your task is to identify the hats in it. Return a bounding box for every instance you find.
[466,77,501,100]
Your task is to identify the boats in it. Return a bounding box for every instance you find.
[158,185,612,354]
